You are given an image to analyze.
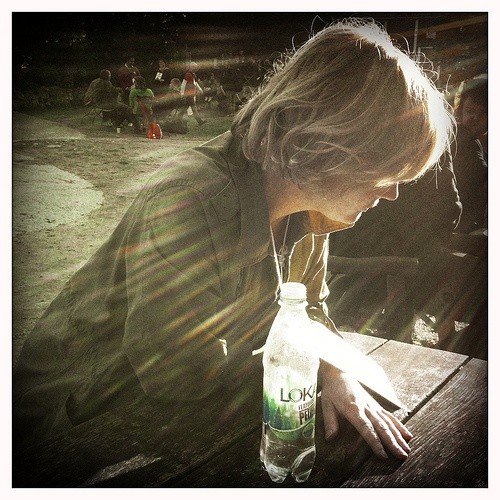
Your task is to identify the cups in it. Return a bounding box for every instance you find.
[117,128,120,133]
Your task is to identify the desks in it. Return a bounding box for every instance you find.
[12,332,489,488]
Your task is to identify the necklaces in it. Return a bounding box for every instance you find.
[277,214,290,278]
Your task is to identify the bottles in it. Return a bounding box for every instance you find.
[259,281,319,483]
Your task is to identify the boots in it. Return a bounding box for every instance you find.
[196,117,207,125]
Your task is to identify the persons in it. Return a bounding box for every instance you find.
[83,68,128,128]
[330,20,464,257]
[118,55,139,96]
[178,61,207,126]
[220,64,244,117]
[197,72,219,102]
[12,20,456,460]
[453,77,488,229]
[152,78,182,120]
[129,76,155,133]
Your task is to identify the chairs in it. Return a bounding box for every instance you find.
[80,93,112,126]
[326,255,419,343]
[132,95,154,129]
